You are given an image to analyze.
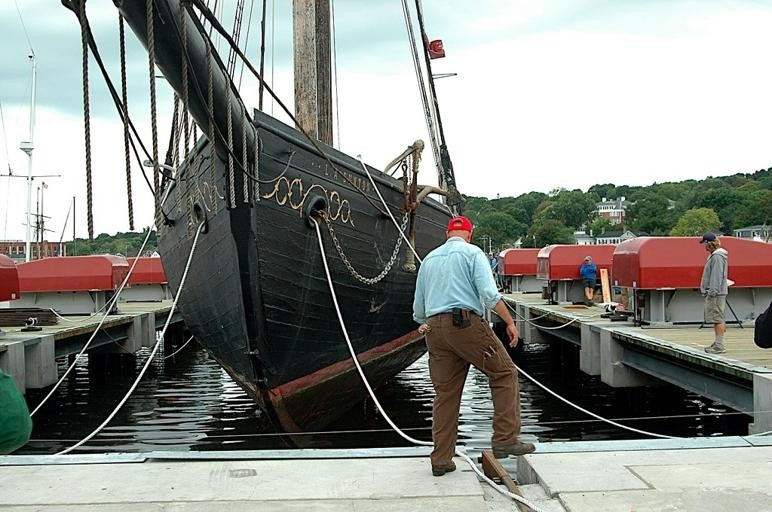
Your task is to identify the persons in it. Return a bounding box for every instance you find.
[579,255,598,301]
[492,246,502,288]
[698,232,729,354]
[505,245,512,249]
[412,216,536,476]
[487,253,497,279]
[485,252,489,260]
[0,369,33,456]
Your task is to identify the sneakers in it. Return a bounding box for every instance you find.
[706,343,727,353]
[432,461,456,476]
[493,439,536,459]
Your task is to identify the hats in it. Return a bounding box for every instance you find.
[700,231,717,243]
[585,256,591,261]
[447,216,473,232]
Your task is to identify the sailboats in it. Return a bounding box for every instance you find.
[60,1,461,449]
